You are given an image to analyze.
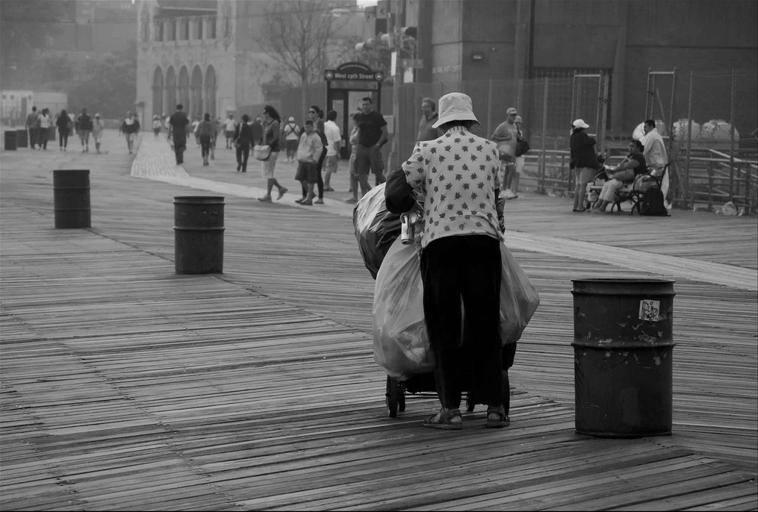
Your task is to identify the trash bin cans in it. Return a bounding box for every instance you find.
[5,131,17,151]
[53,169,92,230]
[49,126,56,141]
[17,130,28,147]
[173,195,225,275]
[569,276,677,437]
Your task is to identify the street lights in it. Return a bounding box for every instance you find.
[331,8,378,22]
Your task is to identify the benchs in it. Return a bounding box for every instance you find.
[585,162,672,215]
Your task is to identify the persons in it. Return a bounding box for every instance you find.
[75,107,92,153]
[589,137,608,180]
[569,119,589,211]
[346,113,372,205]
[294,121,323,206]
[323,110,343,192]
[346,96,388,204]
[26,106,40,149]
[513,115,522,195]
[223,113,237,150]
[152,112,161,136]
[92,112,104,155]
[416,97,443,143]
[490,107,519,199]
[46,108,51,118]
[192,117,200,132]
[257,105,288,202]
[585,140,646,214]
[642,119,672,210]
[384,93,511,432]
[283,116,300,163]
[308,105,328,205]
[55,109,73,151]
[121,110,140,155]
[194,113,214,167]
[168,104,189,165]
[234,114,254,173]
[37,108,51,151]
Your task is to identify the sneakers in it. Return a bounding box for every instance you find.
[314,200,323,204]
[592,209,603,214]
[498,191,511,200]
[586,207,592,213]
[257,193,272,202]
[294,199,303,203]
[507,189,518,200]
[311,192,316,199]
[300,200,312,205]
[276,188,288,201]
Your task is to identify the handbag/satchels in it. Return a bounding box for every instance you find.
[615,159,635,181]
[253,145,272,160]
[515,137,530,157]
[231,123,242,151]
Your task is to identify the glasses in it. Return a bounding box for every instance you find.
[308,110,316,114]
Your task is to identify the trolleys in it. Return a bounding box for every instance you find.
[384,198,511,417]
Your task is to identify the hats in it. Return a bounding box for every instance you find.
[573,119,589,129]
[432,93,480,129]
[506,108,517,115]
[288,117,294,122]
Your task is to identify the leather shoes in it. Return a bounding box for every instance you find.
[576,207,586,212]
[345,199,357,203]
[573,208,576,212]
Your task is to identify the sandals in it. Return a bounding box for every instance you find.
[423,408,462,429]
[485,406,510,427]
[325,186,334,191]
